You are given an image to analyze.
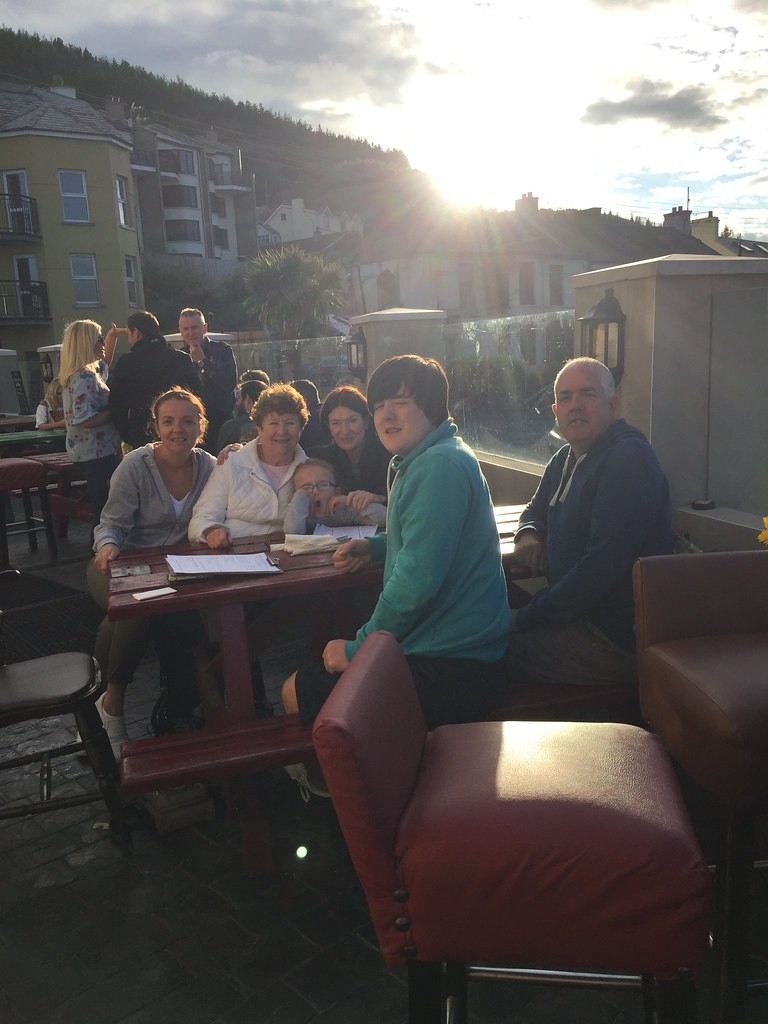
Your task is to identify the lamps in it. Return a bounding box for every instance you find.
[345,327,367,383]
[577,288,627,389]
[39,354,54,384]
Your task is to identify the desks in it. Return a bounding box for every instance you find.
[107,491,556,871]
[0,405,96,540]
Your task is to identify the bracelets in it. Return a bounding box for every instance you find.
[197,360,205,367]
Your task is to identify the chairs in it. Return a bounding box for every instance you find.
[633,548,768,904]
[309,629,714,1024]
[0,457,141,893]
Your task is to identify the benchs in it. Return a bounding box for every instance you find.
[121,709,343,871]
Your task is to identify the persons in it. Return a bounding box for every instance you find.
[281,356,511,803]
[35,308,392,760]
[496,359,672,687]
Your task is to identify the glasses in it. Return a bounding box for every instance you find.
[97,334,105,343]
[299,481,337,494]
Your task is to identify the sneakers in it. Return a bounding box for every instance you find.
[95,691,132,763]
[283,763,332,805]
